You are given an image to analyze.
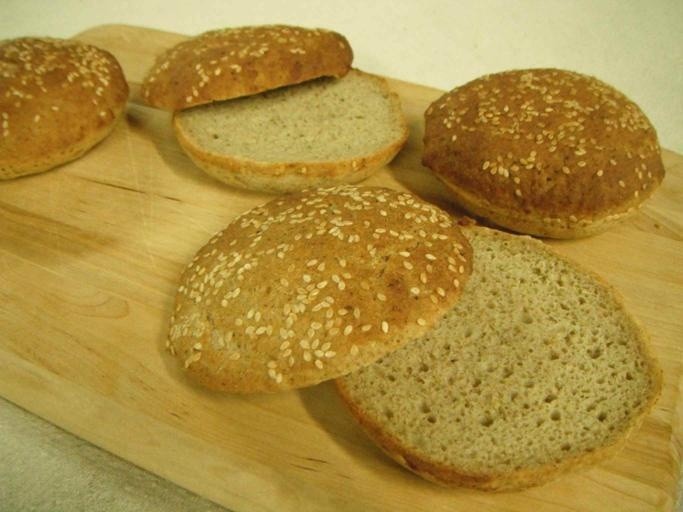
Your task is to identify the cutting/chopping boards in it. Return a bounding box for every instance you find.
[3,23,683,509]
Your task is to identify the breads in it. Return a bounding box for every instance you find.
[139,26,409,194]
[0,37,129,180]
[162,184,664,491]
[421,66,666,239]
